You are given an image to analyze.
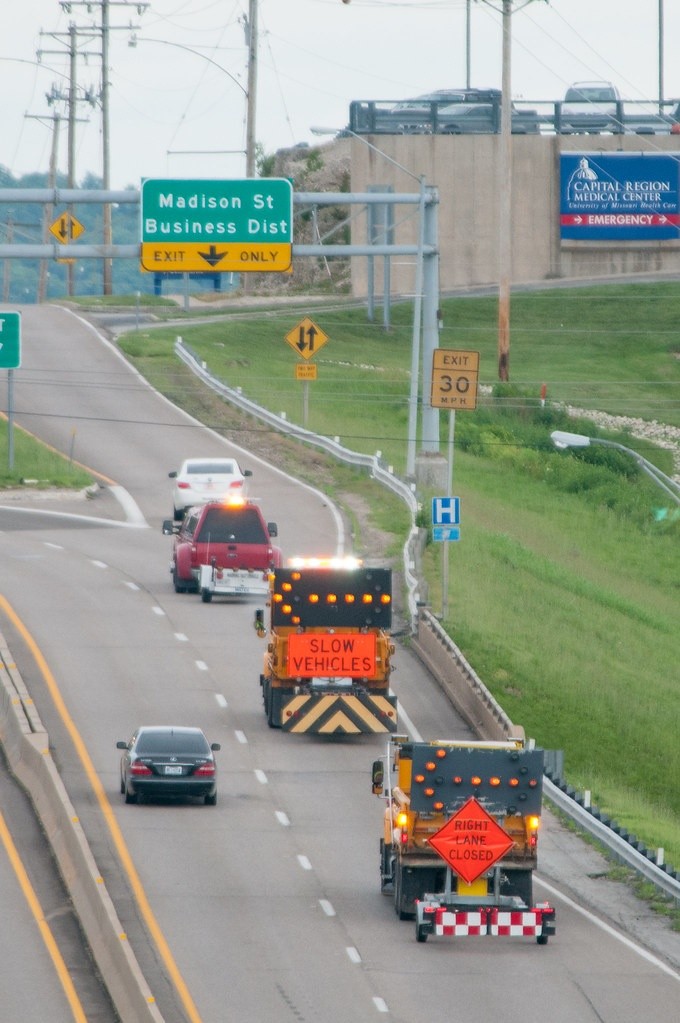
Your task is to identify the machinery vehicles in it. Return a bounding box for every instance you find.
[254,557,397,735]
[371,735,555,945]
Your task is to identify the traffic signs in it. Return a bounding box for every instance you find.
[142,177,294,272]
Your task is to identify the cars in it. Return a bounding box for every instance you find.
[162,458,283,603]
[392,89,526,134]
[117,726,220,805]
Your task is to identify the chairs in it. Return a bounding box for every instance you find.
[599,91,611,100]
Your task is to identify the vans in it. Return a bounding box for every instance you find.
[560,81,625,135]
